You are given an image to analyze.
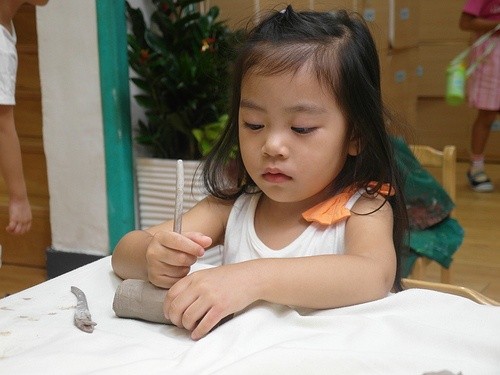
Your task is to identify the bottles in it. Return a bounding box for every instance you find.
[444,57,467,107]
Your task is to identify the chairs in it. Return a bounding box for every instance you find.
[406,144,459,285]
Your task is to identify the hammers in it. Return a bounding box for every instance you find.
[113,158,234,332]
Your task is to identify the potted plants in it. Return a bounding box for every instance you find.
[122,1,244,230]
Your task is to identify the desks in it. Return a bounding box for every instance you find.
[0,254,500,375]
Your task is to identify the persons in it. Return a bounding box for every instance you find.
[459,0,500,192]
[0,0,48,234]
[111,5,412,341]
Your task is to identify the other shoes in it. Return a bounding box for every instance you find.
[467,169,493,191]
